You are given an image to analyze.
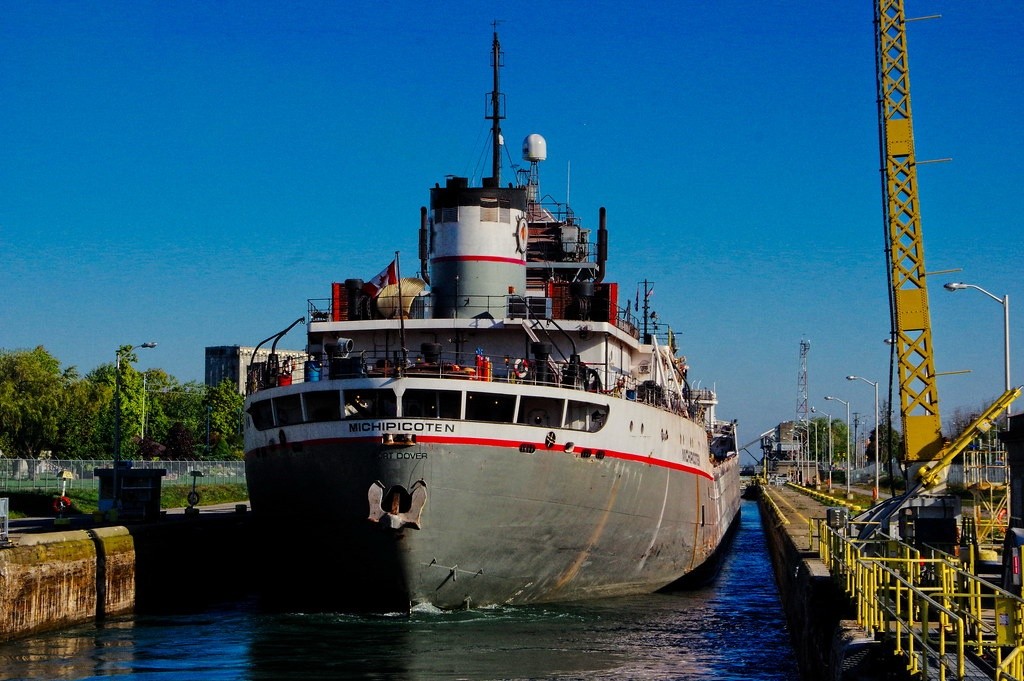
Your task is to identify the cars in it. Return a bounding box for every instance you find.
[30,462,79,480]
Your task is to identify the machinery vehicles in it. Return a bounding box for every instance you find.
[847,0,1023,604]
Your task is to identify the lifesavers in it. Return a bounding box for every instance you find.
[513,359,529,378]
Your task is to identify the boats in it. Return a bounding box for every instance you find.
[740,475,761,498]
[236,20,742,611]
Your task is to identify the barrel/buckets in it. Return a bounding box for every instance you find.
[626,390,635,400]
[278,373,292,386]
[509,287,514,294]
[304,361,322,383]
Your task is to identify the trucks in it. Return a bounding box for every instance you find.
[741,464,764,475]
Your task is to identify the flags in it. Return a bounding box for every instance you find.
[363,259,398,299]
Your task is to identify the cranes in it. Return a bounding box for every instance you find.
[796,335,811,419]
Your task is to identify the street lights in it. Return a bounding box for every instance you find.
[111,341,159,504]
[846,375,882,499]
[824,395,853,500]
[810,407,836,494]
[782,418,822,491]
[885,337,931,417]
[944,279,1013,432]
